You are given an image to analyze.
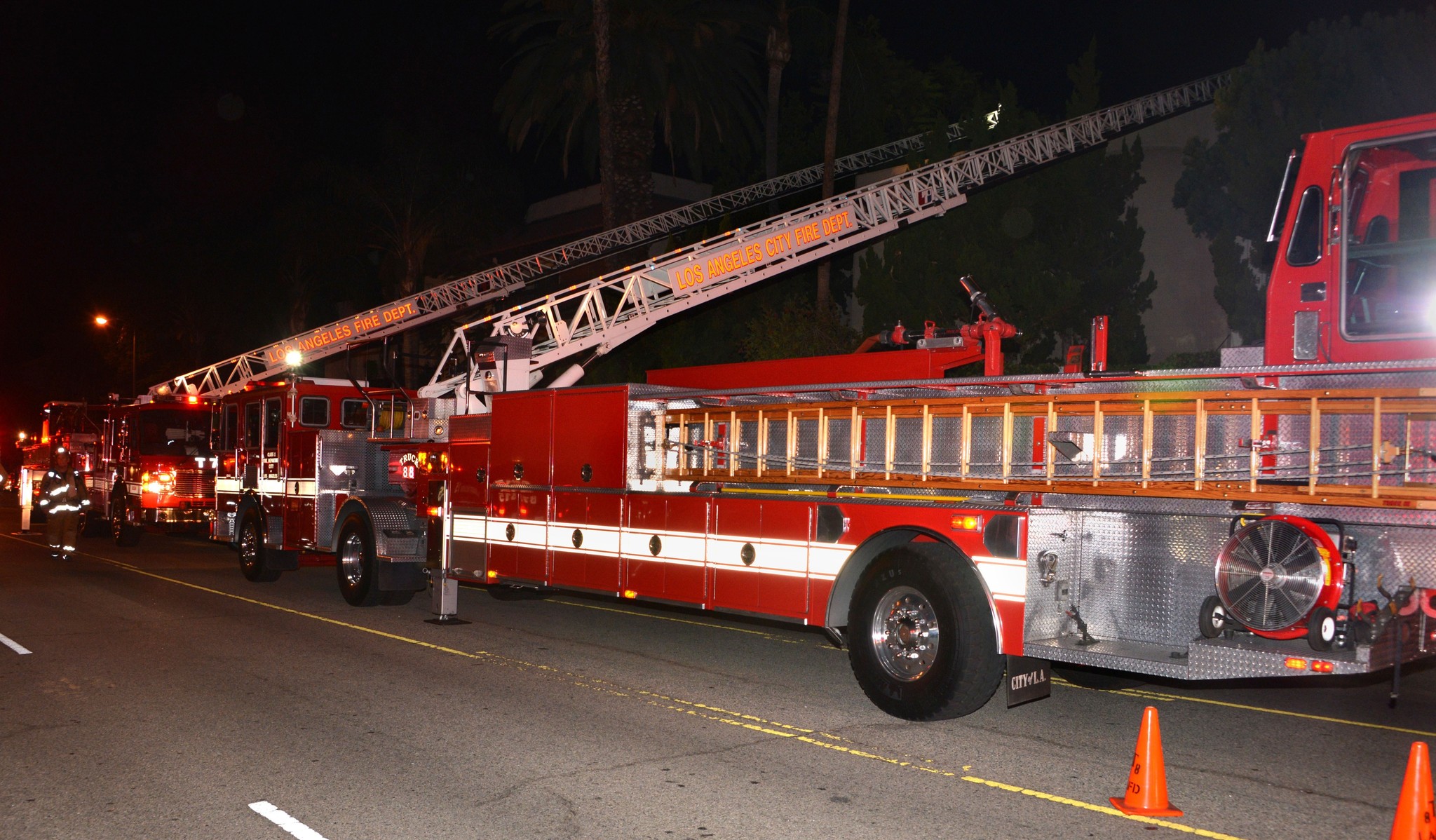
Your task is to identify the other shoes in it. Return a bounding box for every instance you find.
[51,551,59,557]
[62,551,73,559]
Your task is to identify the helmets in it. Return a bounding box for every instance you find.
[54,446,69,456]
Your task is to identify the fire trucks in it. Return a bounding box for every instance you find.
[16,101,1436,723]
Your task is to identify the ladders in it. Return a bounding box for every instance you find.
[663,387,1436,511]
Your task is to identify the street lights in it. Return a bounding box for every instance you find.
[94,316,138,395]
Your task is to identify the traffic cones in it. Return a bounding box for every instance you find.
[1109,706,1185,818]
[1388,741,1436,840]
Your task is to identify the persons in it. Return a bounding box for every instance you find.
[39,447,88,562]
